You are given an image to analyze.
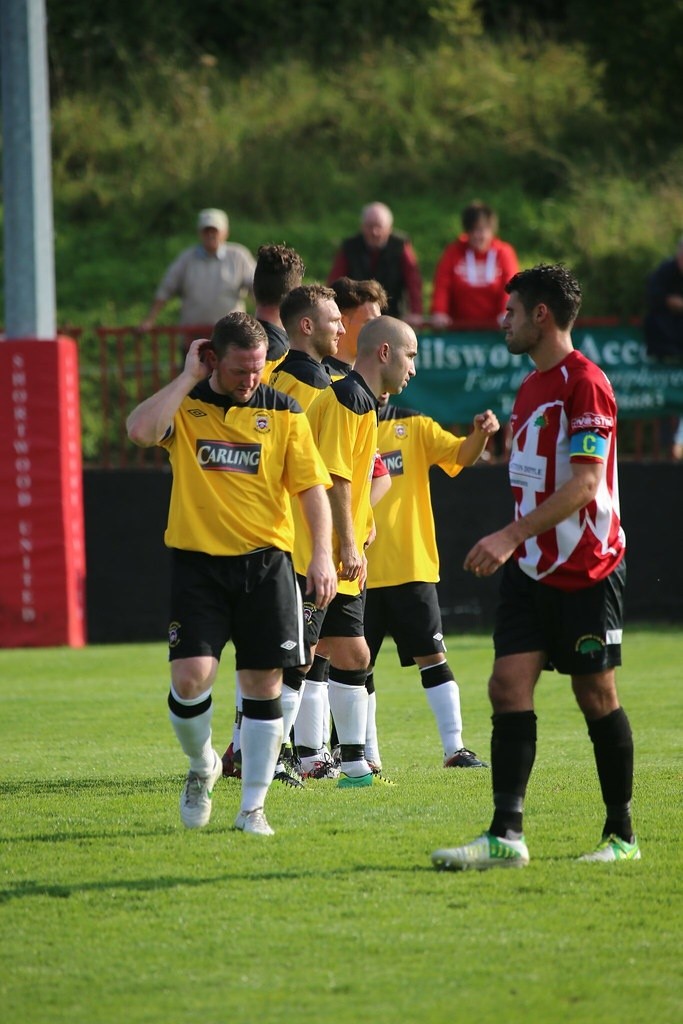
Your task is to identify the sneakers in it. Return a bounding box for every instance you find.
[231,807,275,839]
[221,736,406,795]
[572,833,641,869]
[272,771,308,793]
[178,748,223,829]
[442,748,490,769]
[431,829,530,870]
[336,767,402,792]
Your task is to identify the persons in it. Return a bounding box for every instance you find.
[671,419,683,463]
[430,205,520,330]
[486,423,512,463]
[361,384,500,766]
[221,243,418,790]
[430,266,642,867]
[646,237,683,361]
[326,200,425,332]
[124,312,338,834]
[140,208,257,369]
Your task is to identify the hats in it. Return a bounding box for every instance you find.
[196,208,229,233]
